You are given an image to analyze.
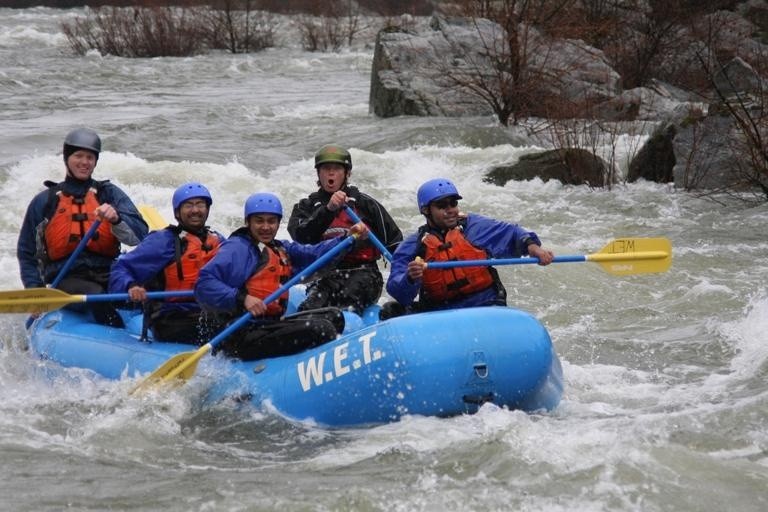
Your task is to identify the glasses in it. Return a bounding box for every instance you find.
[430,199,458,209]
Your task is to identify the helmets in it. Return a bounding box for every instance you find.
[314,144,351,167]
[65,128,101,153]
[245,193,283,221]
[172,182,212,208]
[418,178,462,213]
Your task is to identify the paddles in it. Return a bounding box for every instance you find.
[126,232,361,398]
[138,205,168,231]
[0,288,196,313]
[415,238,673,274]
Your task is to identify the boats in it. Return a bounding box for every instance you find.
[22,283,563,418]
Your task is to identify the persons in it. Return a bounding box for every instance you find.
[107,181,227,350]
[281,143,403,313]
[194,191,369,362]
[379,175,553,320]
[15,126,150,330]
